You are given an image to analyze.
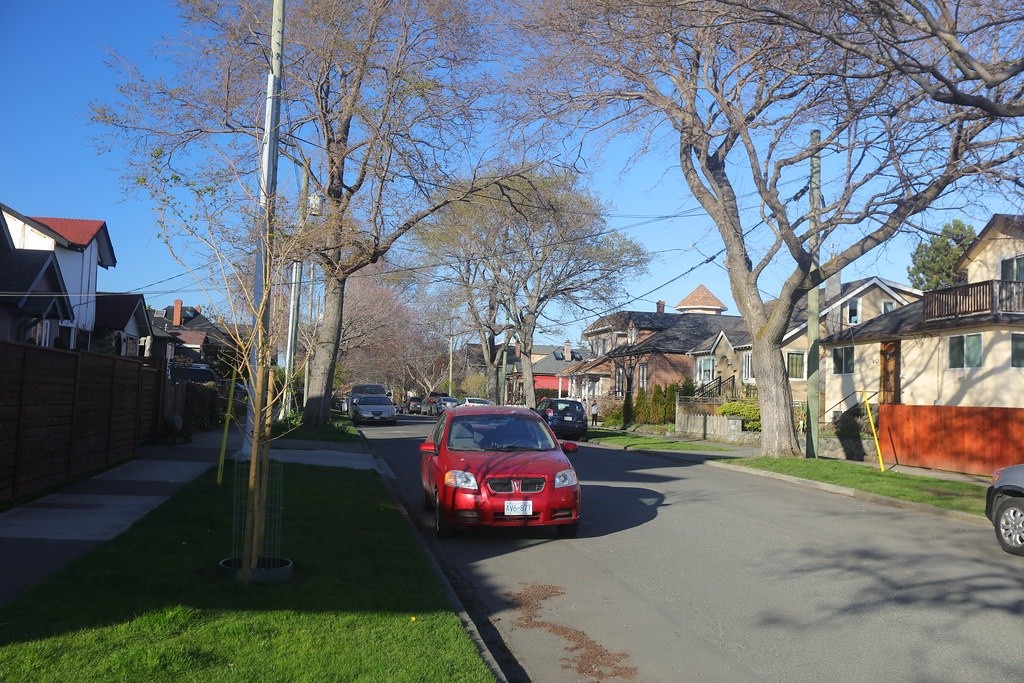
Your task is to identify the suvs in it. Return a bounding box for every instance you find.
[403,397,423,414]
[421,391,450,416]
[347,384,392,420]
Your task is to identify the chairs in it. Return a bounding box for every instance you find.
[452,422,479,448]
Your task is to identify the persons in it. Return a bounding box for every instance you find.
[591,400,598,427]
[582,398,587,410]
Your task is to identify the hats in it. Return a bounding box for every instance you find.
[592,399,596,404]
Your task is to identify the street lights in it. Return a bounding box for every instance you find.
[427,331,453,398]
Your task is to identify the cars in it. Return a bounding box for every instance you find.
[352,394,397,425]
[530,398,588,439]
[432,397,458,416]
[455,398,491,408]
[984,464,1024,555]
[419,406,583,538]
[398,404,403,414]
